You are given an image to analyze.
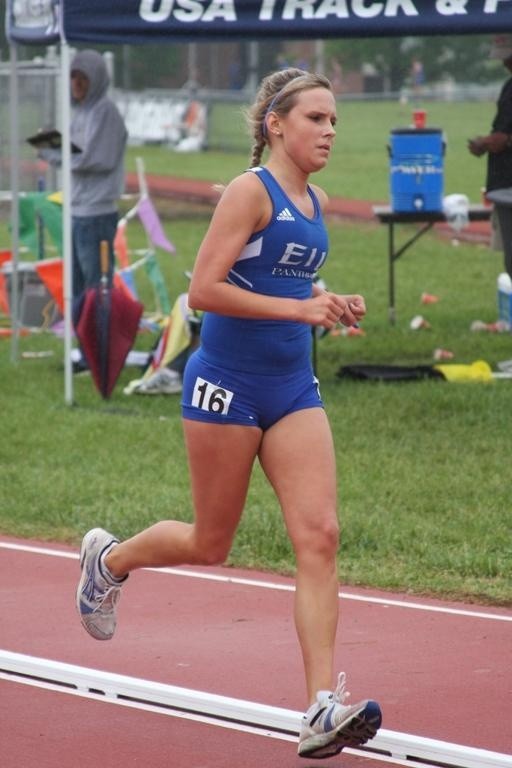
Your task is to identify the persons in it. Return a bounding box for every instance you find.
[74,64,385,762]
[466,53,512,279]
[29,47,132,379]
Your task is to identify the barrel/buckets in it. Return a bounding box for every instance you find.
[495,275,511,324]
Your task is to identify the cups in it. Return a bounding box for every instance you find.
[434,347,454,362]
[413,111,426,130]
[421,293,438,303]
[408,316,430,331]
[481,187,491,207]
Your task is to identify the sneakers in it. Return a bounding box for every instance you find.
[126,367,185,398]
[295,672,383,760]
[71,524,131,644]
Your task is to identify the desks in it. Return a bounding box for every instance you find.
[375,211,492,307]
